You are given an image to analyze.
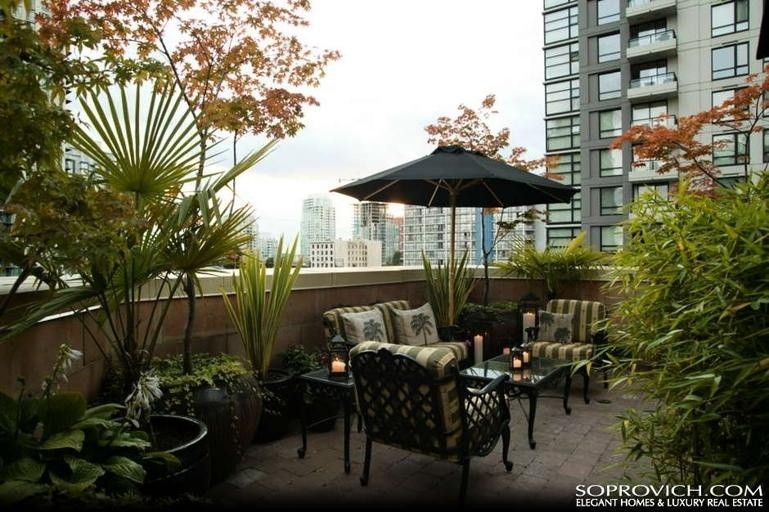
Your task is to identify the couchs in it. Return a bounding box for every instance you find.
[326,300,468,380]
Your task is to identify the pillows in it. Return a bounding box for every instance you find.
[536,309,574,343]
[342,309,388,345]
[390,302,440,348]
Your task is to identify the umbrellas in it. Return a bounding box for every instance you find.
[328,143,581,324]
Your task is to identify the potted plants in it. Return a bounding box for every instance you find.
[421,247,486,342]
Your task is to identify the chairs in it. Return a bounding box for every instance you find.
[348,341,513,511]
[525,300,606,404]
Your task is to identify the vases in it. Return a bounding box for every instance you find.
[492,301,521,343]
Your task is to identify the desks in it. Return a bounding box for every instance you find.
[297,366,363,475]
[460,354,574,450]
